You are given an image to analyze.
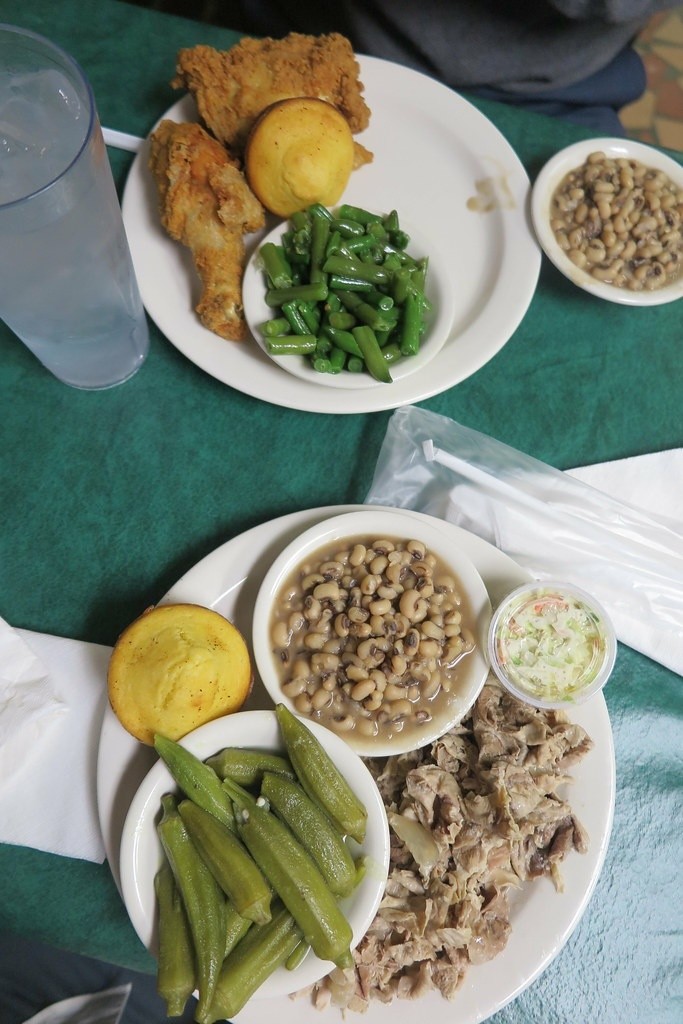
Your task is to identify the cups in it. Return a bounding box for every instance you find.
[1,22,148,390]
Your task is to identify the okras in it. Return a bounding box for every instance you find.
[153,704,372,1024]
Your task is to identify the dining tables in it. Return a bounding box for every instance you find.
[1,0,683,1021]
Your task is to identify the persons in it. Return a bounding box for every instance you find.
[124,0,657,135]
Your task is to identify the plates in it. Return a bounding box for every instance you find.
[248,510,496,759]
[524,134,683,307]
[118,48,547,413]
[237,207,455,390]
[120,709,395,1002]
[90,503,617,1024]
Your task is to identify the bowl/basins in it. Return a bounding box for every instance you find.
[485,577,618,711]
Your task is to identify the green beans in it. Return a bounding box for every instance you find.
[273,539,475,734]
[552,152,683,289]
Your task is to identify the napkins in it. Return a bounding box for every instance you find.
[450,445,683,678]
[0,616,118,869]
